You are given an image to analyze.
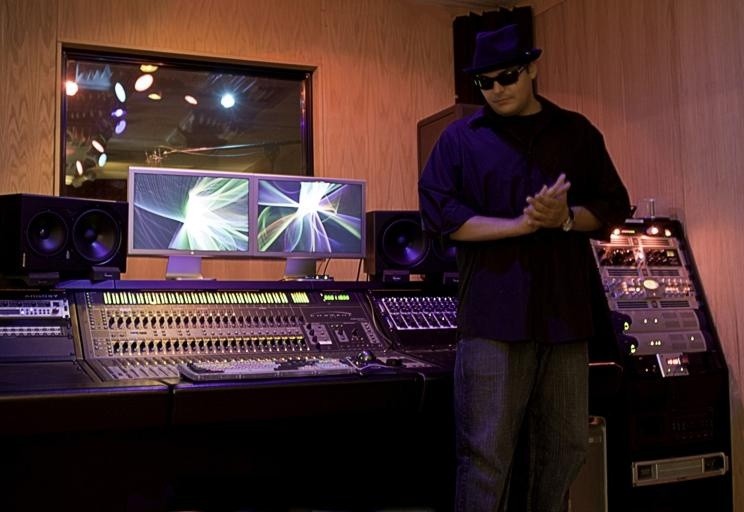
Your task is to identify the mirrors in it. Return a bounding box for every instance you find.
[53,39,318,202]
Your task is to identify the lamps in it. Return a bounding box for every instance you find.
[73,64,159,176]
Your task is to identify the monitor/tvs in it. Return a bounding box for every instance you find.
[128,165,253,282]
[254,173,367,282]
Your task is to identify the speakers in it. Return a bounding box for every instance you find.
[453,5,538,104]
[362,209,461,283]
[0,193,128,282]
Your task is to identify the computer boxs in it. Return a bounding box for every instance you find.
[566,415,610,512]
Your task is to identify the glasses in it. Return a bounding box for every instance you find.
[471,67,527,90]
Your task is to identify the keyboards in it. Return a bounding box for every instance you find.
[176,358,355,382]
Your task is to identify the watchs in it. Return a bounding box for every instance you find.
[559,207,574,233]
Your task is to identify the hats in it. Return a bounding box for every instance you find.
[462,25,542,73]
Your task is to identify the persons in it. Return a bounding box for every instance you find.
[418,24,631,512]
